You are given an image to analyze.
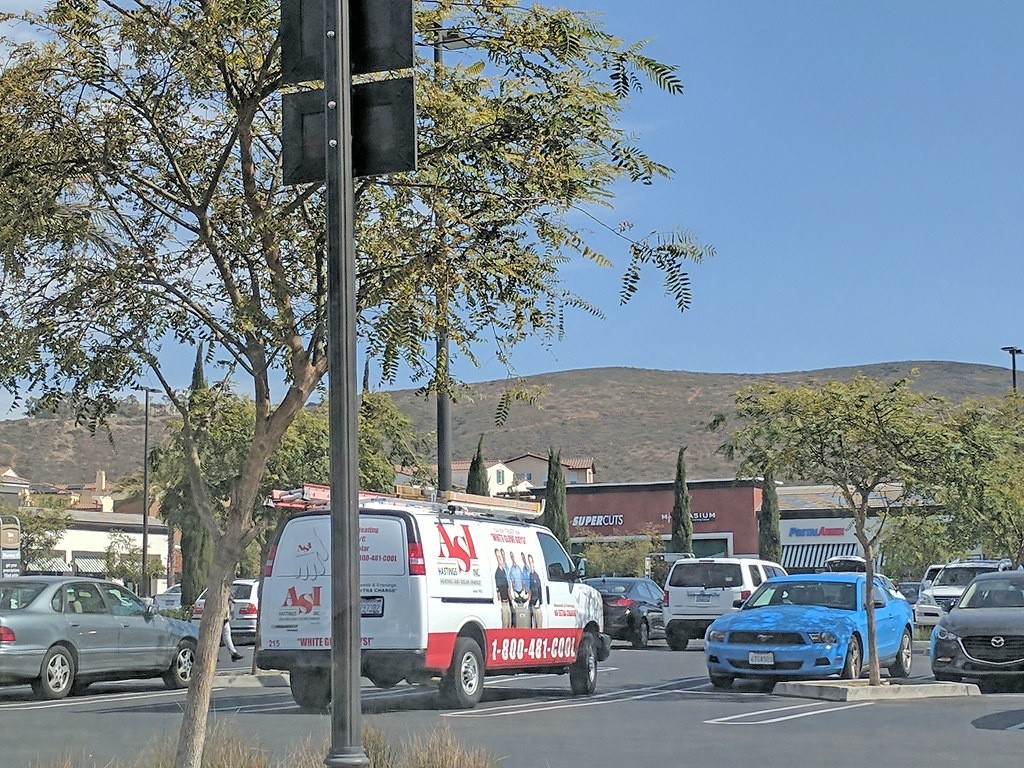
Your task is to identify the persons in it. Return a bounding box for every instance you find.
[495,548,543,629]
[222,605,243,663]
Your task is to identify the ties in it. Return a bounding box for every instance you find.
[502,568,506,576]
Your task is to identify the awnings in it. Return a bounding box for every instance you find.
[781,544,857,568]
[23,557,110,573]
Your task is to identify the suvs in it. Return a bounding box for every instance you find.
[819,555,907,602]
[915,559,1023,630]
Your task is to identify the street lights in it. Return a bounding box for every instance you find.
[1003,347,1023,392]
[132,385,163,597]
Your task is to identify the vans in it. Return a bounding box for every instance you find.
[253,496,610,711]
[919,562,982,604]
[662,557,790,651]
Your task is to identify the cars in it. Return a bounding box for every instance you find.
[930,564,1024,683]
[191,579,259,646]
[897,582,921,604]
[704,571,913,686]
[154,583,182,610]
[0,575,200,700]
[581,578,667,650]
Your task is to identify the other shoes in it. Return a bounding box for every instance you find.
[230,653,244,662]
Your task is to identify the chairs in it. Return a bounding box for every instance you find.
[108,593,127,613]
